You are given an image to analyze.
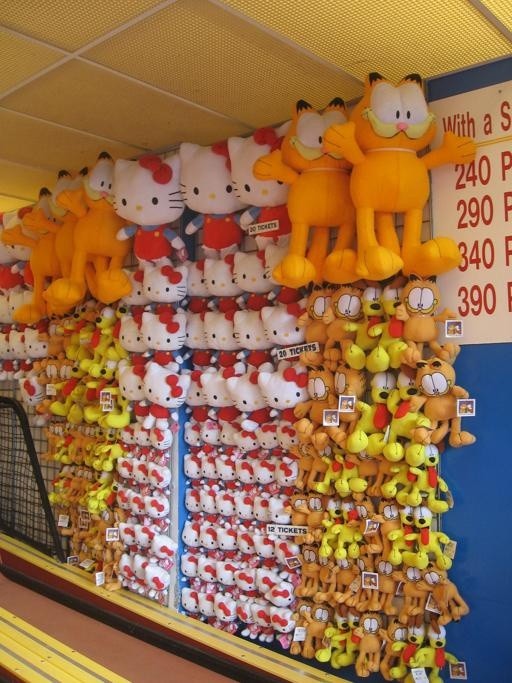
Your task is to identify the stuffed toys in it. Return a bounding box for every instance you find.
[115,151,188,604]
[1,152,129,428]
[1,69,478,683]
[255,72,478,683]
[41,424,127,590]
[180,120,310,649]
